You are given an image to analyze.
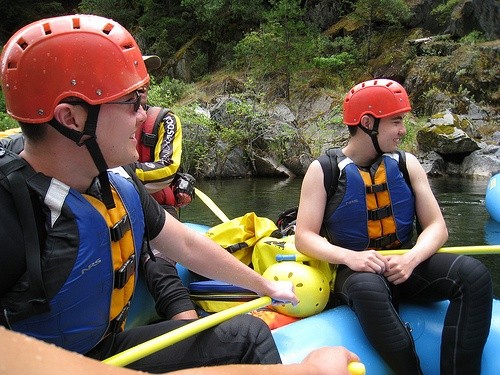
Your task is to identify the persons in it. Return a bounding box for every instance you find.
[294,79,494,375]
[1,14,366,374]
[133,55,198,320]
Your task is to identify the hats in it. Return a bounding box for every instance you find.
[142,55,161,71]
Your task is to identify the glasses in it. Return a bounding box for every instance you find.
[104,89,141,113]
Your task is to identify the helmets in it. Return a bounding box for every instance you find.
[261,261,330,318]
[343,79,411,125]
[0,14,150,123]
